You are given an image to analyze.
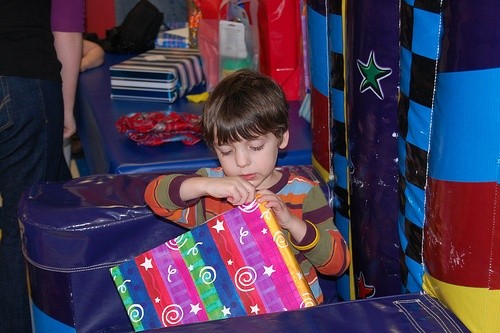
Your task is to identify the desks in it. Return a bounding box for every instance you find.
[74,53,312,174]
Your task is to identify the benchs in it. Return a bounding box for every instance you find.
[16,163,339,333]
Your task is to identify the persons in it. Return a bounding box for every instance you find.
[48,0,104,180]
[0,0,72,333]
[144,69,351,306]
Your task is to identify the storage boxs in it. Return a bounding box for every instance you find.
[110,47,205,103]
[109,193,318,332]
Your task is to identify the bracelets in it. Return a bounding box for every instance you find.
[289,220,319,250]
